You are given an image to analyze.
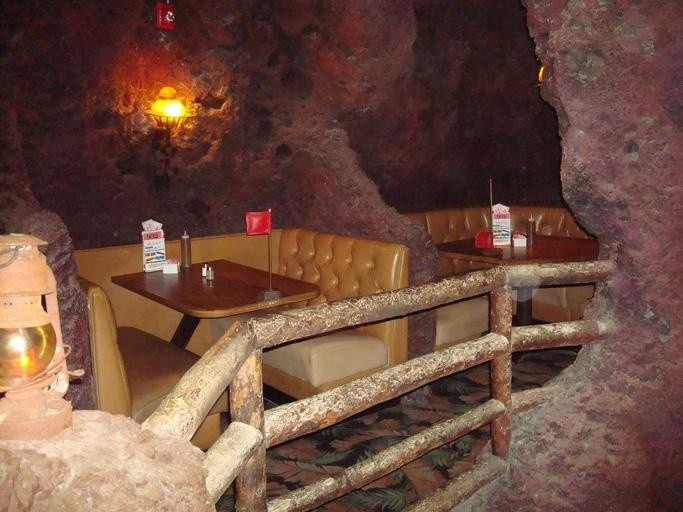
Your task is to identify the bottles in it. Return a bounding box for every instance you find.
[181,231,192,269]
[201,263,215,280]
[528,211,536,244]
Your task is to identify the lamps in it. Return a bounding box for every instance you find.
[146,86,194,138]
[1,231,84,442]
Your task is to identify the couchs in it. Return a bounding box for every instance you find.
[79,275,229,451]
[261,226,409,400]
[402,205,597,351]
